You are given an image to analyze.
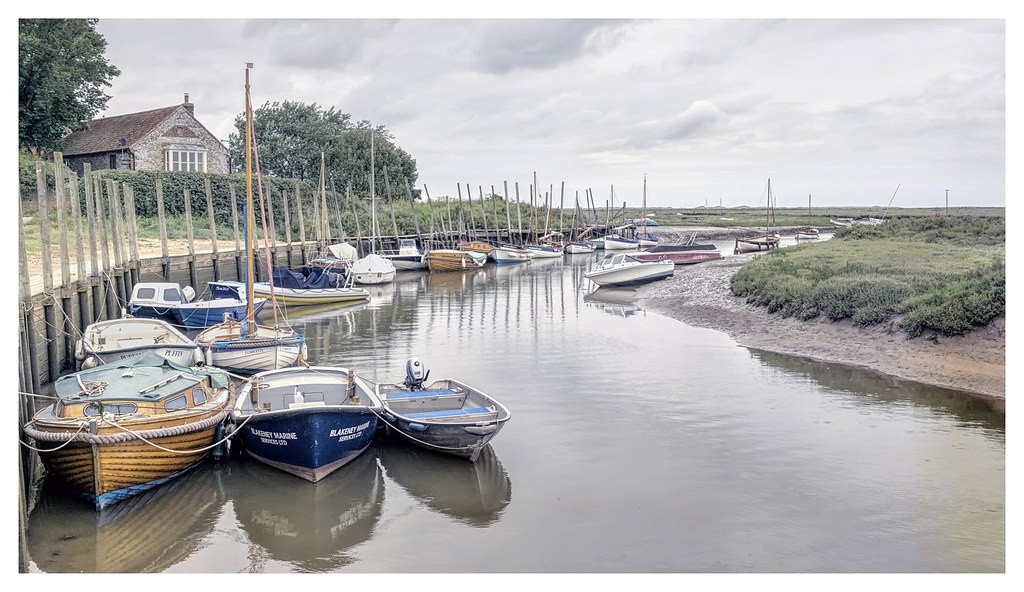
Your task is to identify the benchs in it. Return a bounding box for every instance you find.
[401,406,498,422]
[379,388,466,403]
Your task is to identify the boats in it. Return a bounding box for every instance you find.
[625,232,723,266]
[227,439,387,560]
[25,455,225,574]
[829,184,900,230]
[229,365,382,485]
[583,285,643,318]
[252,264,370,306]
[127,281,267,332]
[584,253,675,286]
[373,437,513,528]
[75,306,199,374]
[258,298,370,330]
[23,351,236,512]
[371,357,512,463]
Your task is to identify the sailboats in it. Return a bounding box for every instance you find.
[305,131,658,285]
[191,62,305,376]
[797,194,819,239]
[734,178,780,254]
